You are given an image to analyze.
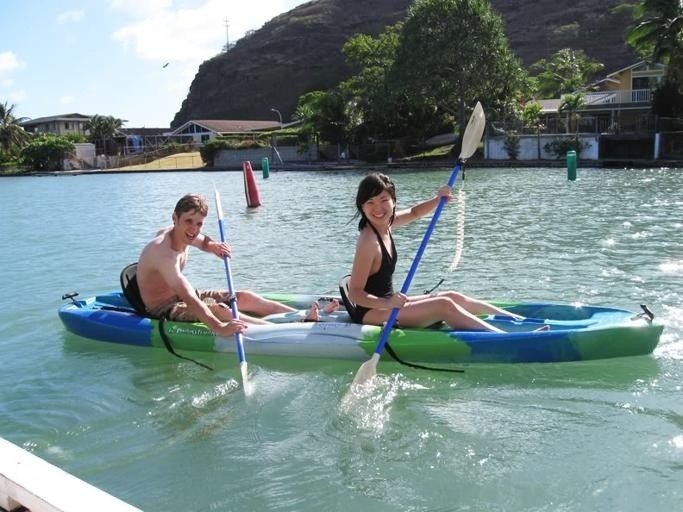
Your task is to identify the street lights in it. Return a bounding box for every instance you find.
[270,108,283,129]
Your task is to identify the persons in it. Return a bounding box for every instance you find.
[135,193,340,337]
[349,172,550,333]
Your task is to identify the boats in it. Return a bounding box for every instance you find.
[55,288,664,366]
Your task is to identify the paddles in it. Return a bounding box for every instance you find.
[342,101,486,403]
[213,189,251,398]
